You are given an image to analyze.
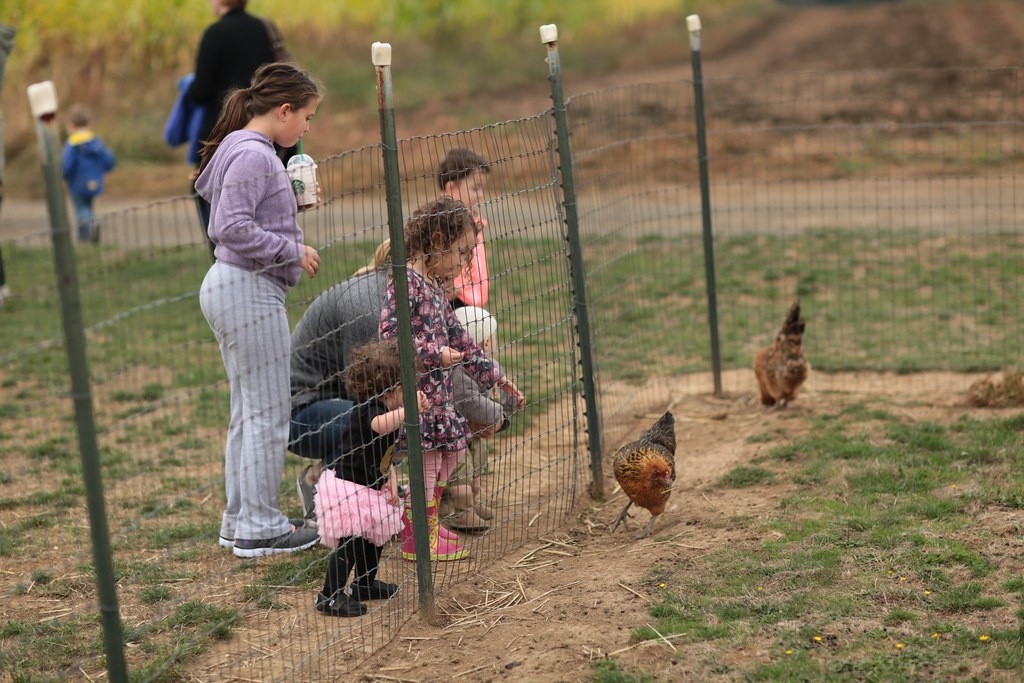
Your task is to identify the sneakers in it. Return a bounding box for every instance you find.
[220,521,320,558]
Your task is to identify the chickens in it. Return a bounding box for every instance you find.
[610,408,677,534]
[965,362,1024,410]
[754,299,810,413]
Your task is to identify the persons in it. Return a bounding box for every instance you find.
[315,340,430,617]
[59,104,115,244]
[289,241,510,528]
[0,25,15,302]
[192,0,300,263]
[436,144,489,307]
[200,62,320,556]
[449,304,497,531]
[378,196,520,562]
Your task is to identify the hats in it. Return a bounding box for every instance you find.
[452,305,498,345]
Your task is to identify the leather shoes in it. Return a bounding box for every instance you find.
[317,591,367,618]
[348,581,399,601]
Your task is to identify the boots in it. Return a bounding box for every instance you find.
[448,486,497,530]
[402,481,469,561]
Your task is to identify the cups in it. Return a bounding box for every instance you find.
[287,153,318,205]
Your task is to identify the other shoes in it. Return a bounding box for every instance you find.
[90,221,101,244]
